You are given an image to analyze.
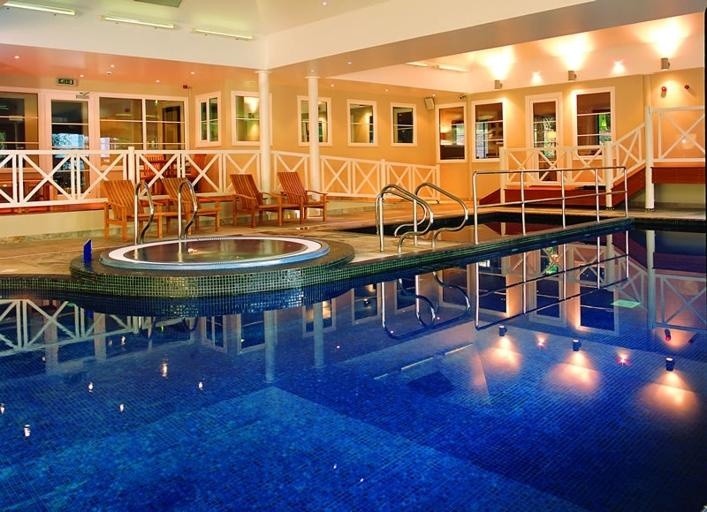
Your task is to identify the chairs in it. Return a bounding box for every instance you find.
[104,172,329,242]
[144,154,165,176]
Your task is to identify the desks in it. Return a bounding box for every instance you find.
[0,172,52,203]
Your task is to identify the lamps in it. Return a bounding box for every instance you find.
[103,14,174,29]
[661,58,670,70]
[665,358,675,371]
[1,1,75,16]
[572,340,581,351]
[499,326,507,336]
[568,70,576,80]
[495,80,502,89]
[194,27,252,39]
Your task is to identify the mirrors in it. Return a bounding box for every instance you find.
[390,102,417,147]
[471,97,508,163]
[571,86,616,160]
[297,96,331,147]
[347,99,378,147]
[231,91,273,146]
[435,102,468,164]
